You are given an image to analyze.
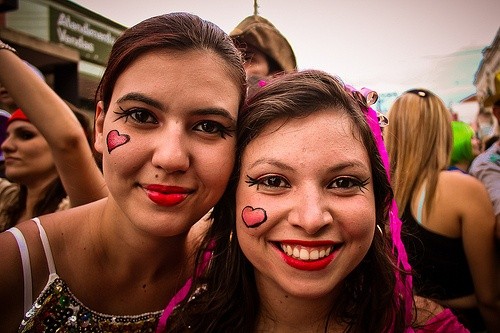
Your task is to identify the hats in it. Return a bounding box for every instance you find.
[5,108,32,130]
[228,14,297,73]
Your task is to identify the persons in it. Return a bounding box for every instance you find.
[383,89,500,333]
[0,40,109,234]
[156,69,470,333]
[229,15,298,88]
[444,72,500,250]
[0,12,246,333]
